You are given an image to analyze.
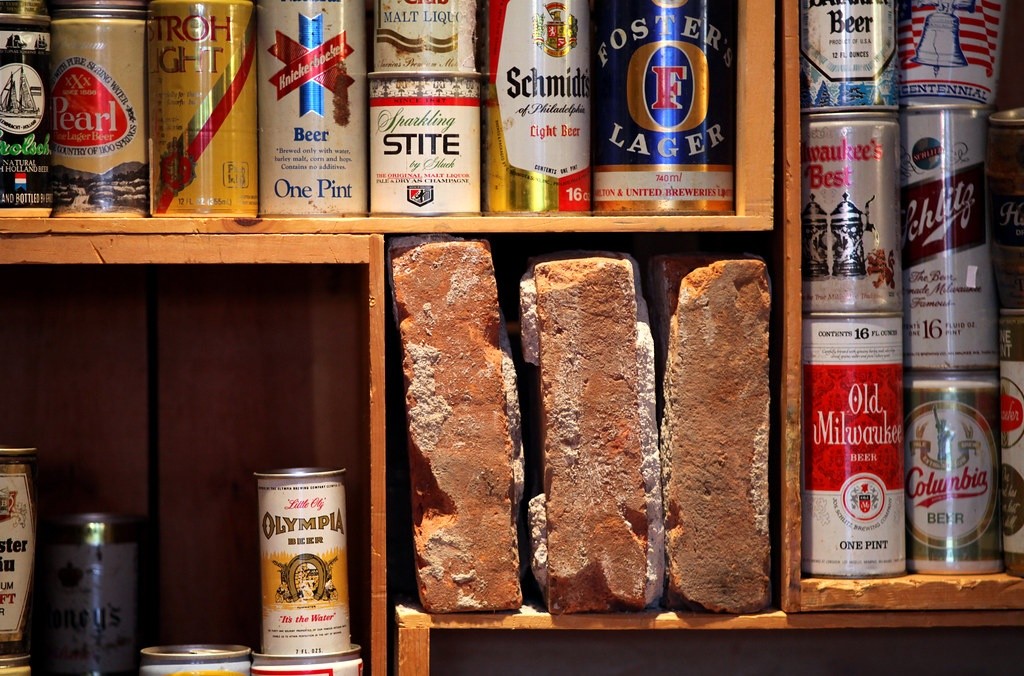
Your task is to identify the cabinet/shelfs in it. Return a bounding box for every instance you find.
[0,0,1023,676]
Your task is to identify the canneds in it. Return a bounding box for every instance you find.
[0,0,738,217]
[795,0,1024,579]
[0,449,364,676]
